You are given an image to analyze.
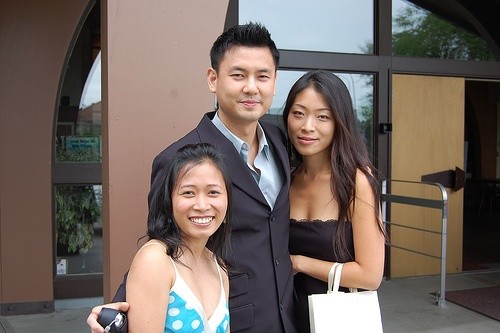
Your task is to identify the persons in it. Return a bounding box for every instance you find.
[283,69,389,333]
[86,22,293,333]
[125,142,239,333]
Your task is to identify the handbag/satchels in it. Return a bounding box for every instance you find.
[308,262,383,333]
